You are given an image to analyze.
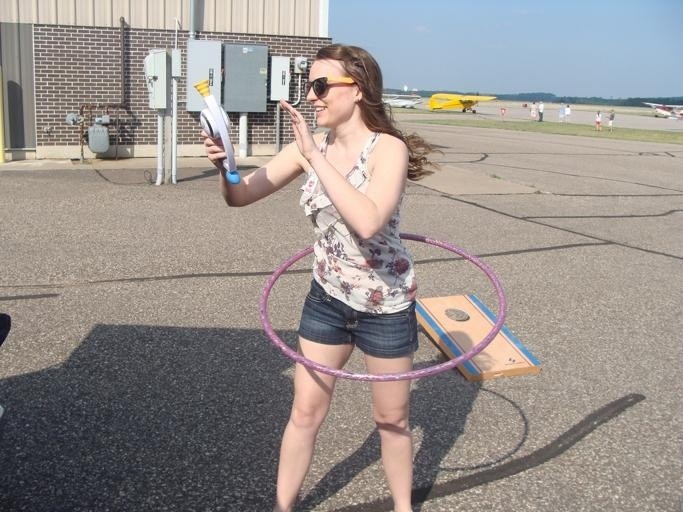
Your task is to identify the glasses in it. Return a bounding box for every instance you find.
[303,75,355,99]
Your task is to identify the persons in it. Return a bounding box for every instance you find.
[556,102,565,122]
[564,105,570,124]
[529,102,537,121]
[608,109,615,132]
[199,42,418,511]
[595,111,601,131]
[537,101,544,122]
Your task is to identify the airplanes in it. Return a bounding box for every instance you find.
[428,93,497,114]
[642,101,682,119]
[381,93,422,109]
